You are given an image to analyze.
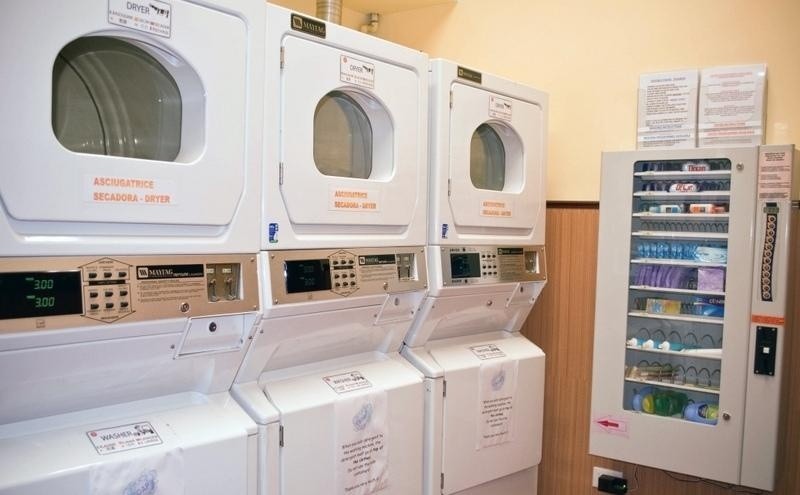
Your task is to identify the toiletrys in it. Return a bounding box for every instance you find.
[626,337,702,352]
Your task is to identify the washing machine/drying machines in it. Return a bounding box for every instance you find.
[0,0,547,495]
